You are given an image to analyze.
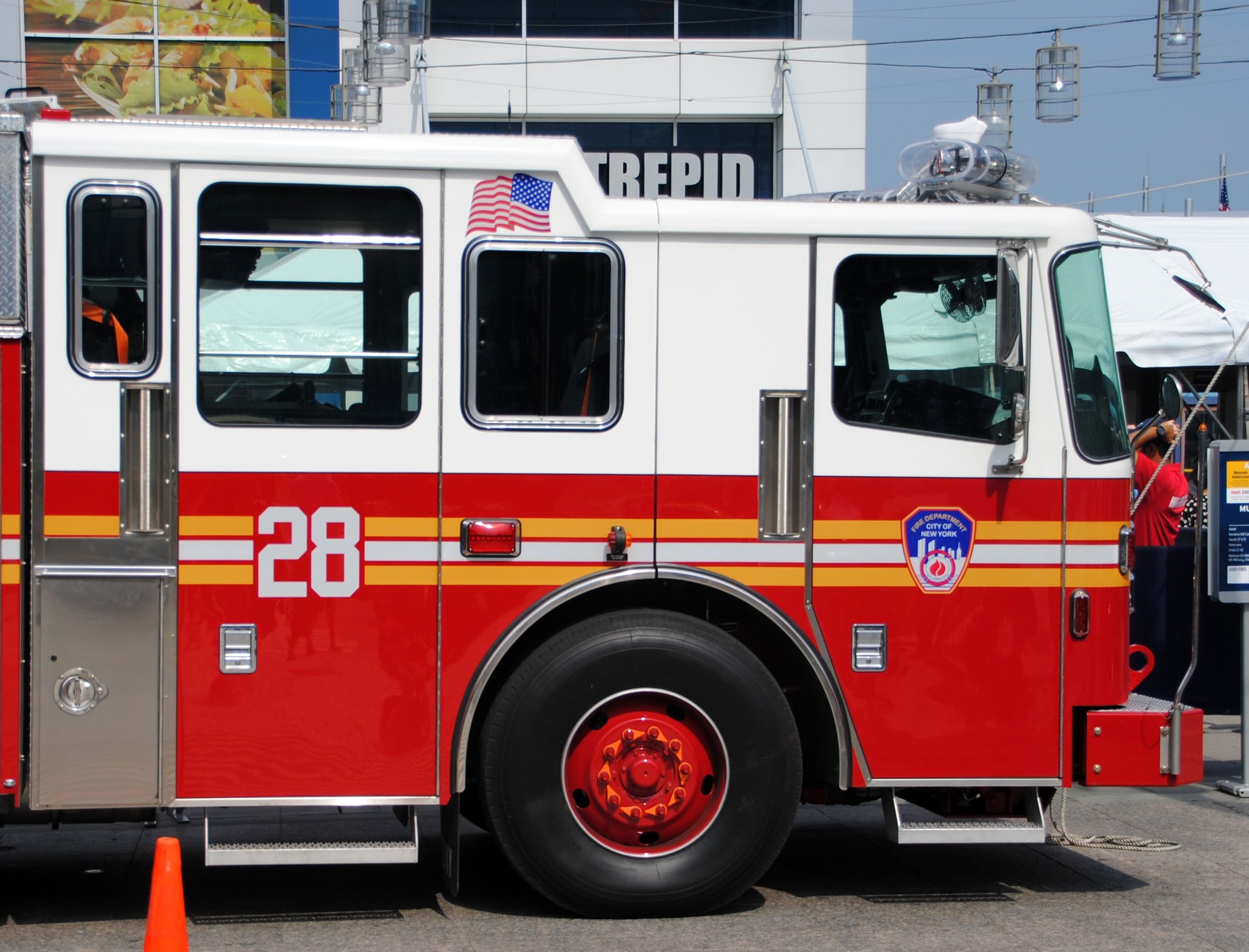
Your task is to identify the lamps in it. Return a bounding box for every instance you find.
[1036,27,1077,123]
[976,65,1014,148]
[330,0,428,124]
[1153,2,1202,80]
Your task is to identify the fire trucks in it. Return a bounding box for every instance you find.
[0,82,1230,921]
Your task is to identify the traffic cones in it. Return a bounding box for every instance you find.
[139,834,193,952]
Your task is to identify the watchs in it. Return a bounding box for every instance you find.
[1156,424,1165,439]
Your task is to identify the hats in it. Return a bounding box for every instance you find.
[1128,419,1166,433]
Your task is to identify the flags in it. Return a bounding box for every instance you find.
[1219,175,1230,211]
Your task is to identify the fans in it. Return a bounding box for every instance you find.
[941,275,996,323]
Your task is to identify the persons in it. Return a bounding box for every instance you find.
[1179,456,1208,528]
[1127,417,1189,698]
